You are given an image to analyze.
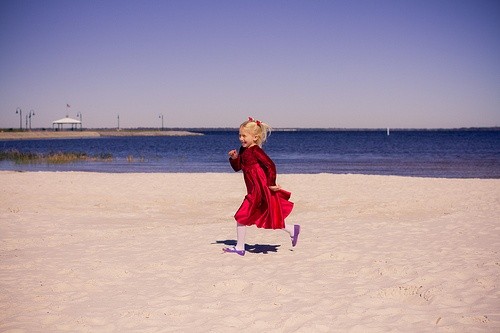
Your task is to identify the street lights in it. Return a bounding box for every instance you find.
[76,112,83,131]
[29,111,35,130]
[65,103,74,130]
[16,107,22,130]
[158,113,163,128]
[26,114,30,131]
[117,115,120,129]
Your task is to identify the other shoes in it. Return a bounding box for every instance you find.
[222,246,245,255]
[290,224,300,246]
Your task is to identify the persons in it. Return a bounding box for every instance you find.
[223,116,300,257]
